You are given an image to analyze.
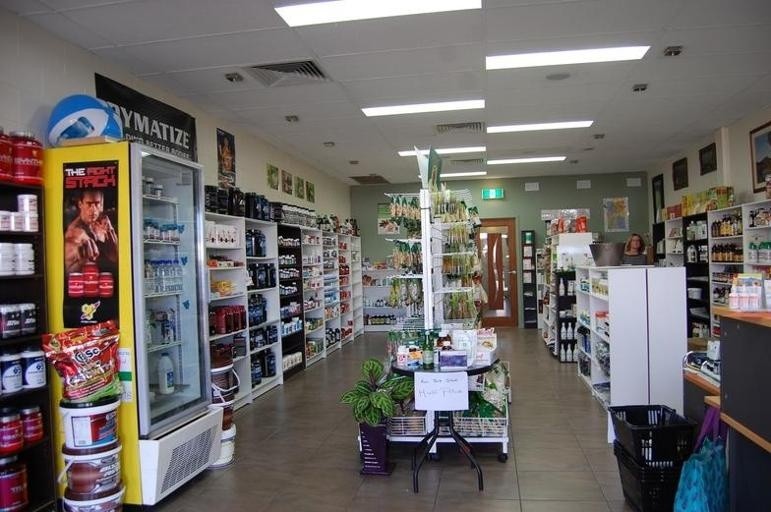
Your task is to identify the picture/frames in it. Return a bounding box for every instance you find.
[671,156,689,192]
[697,142,717,176]
[651,173,665,223]
[748,121,771,193]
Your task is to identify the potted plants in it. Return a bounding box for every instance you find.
[338,357,416,477]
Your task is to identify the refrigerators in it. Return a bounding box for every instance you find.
[46,142,223,505]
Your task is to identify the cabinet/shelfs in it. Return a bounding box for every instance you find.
[0,181,60,511]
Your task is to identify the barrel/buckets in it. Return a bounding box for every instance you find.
[210,356,241,403]
[208,399,236,432]
[588,240,627,266]
[59,394,122,450]
[207,423,237,468]
[57,443,123,495]
[61,484,126,511]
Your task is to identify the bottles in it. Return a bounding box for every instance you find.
[367,299,397,325]
[559,277,565,296]
[143,259,184,293]
[143,176,164,198]
[143,216,181,242]
[691,323,710,338]
[144,309,175,346]
[711,212,743,304]
[279,268,300,295]
[560,322,578,362]
[158,351,176,395]
[205,186,276,392]
[406,329,451,370]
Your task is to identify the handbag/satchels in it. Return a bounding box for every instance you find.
[672,407,727,512]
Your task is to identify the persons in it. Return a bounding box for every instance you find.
[622,233,646,265]
[65,189,119,273]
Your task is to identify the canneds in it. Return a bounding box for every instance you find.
[0,193,38,232]
[68,262,115,297]
[1,406,22,454]
[21,405,44,444]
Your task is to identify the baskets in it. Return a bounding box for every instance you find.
[608,405,698,512]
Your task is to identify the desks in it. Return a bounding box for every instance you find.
[390,355,501,493]
